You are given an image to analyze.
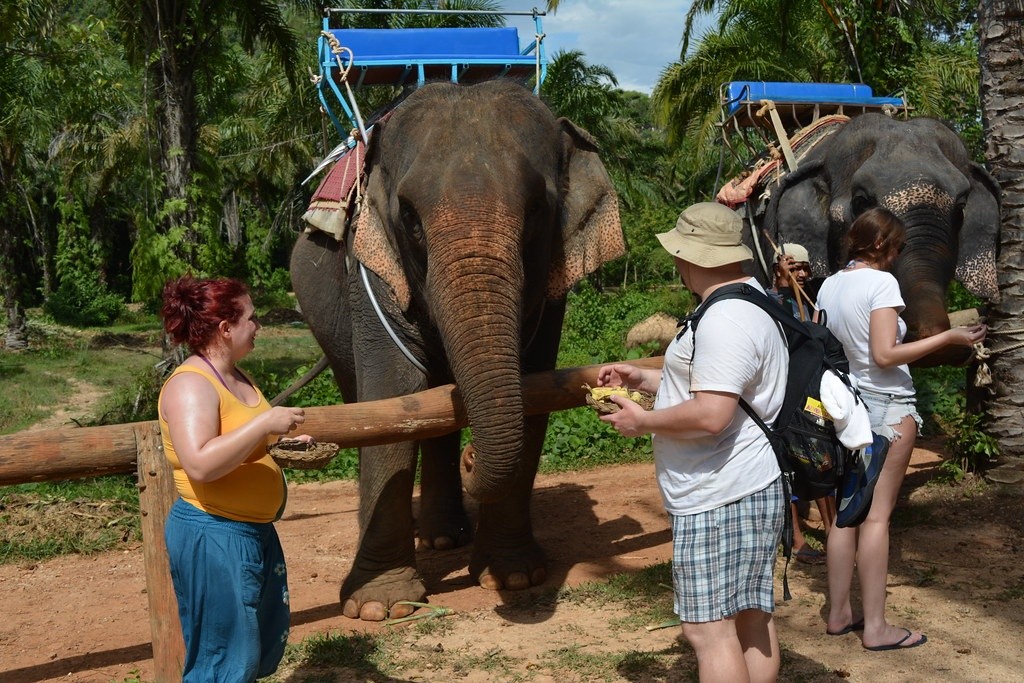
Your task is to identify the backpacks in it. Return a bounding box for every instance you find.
[686,282,872,501]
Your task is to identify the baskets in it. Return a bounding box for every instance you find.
[266,439,340,470]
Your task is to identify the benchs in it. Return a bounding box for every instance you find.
[314,8,550,141]
[718,81,914,175]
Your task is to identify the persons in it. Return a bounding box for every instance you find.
[597,201,790,683]
[812,208,987,650]
[157,279,314,683]
[766,242,836,565]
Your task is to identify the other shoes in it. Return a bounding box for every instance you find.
[835,429,889,529]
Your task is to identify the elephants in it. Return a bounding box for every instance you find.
[289,71,626,620]
[715,112,1001,522]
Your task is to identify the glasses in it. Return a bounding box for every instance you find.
[893,239,907,254]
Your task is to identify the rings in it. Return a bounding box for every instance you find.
[782,259,786,260]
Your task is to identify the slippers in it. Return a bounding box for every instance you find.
[865,628,927,651]
[792,548,825,565]
[827,618,865,634]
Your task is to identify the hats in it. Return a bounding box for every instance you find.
[654,201,754,268]
[773,242,809,263]
